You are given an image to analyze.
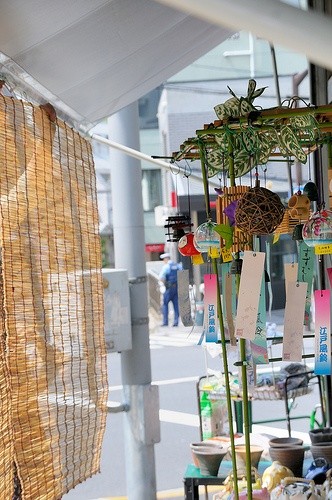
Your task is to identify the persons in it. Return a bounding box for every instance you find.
[158,254,184,327]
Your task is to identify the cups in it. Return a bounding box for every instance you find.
[309,428,332,445]
[268,437,304,447]
[192,449,227,476]
[269,445,304,478]
[191,443,223,469]
[228,443,264,477]
[309,442,332,469]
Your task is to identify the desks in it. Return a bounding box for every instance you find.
[184,459,313,500]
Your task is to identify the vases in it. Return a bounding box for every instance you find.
[269,438,305,482]
[190,442,264,476]
[310,442,332,468]
[309,427,332,443]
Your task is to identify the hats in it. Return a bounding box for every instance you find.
[160,253,171,259]
